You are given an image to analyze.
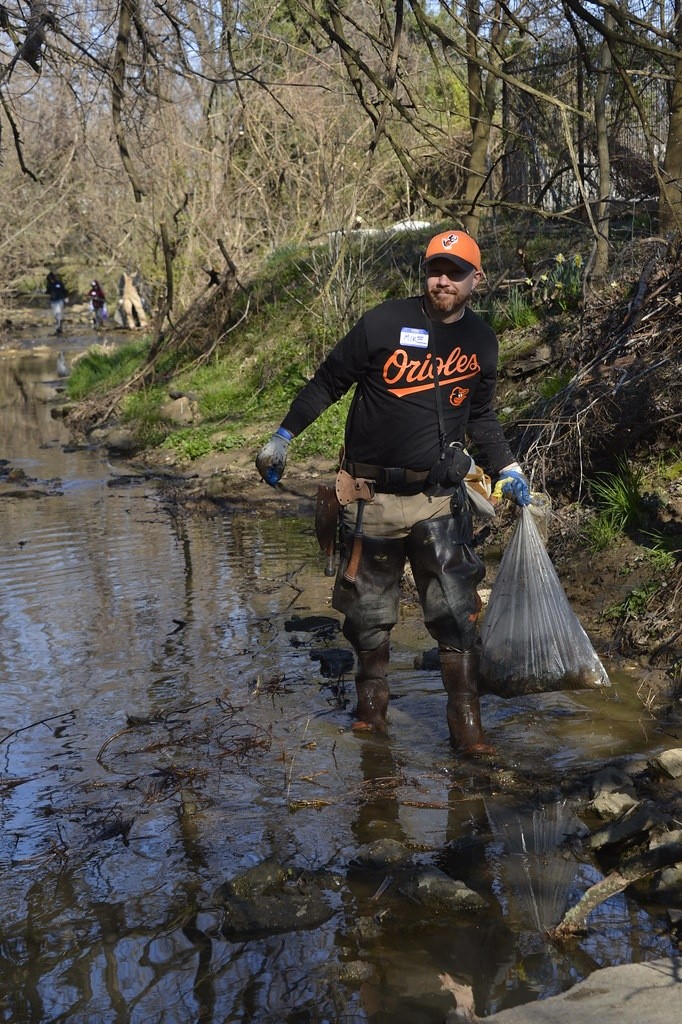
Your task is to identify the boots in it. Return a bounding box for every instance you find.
[351,635,392,732]
[438,649,500,756]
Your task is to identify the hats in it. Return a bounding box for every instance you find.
[422,230,482,275]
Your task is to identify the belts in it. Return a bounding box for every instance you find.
[344,461,429,486]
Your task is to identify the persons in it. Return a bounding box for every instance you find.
[255,229,532,769]
[45,272,71,334]
[87,280,107,331]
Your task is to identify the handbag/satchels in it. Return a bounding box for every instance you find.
[429,446,472,489]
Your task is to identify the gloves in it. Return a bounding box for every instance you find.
[254,434,291,486]
[500,462,531,506]
